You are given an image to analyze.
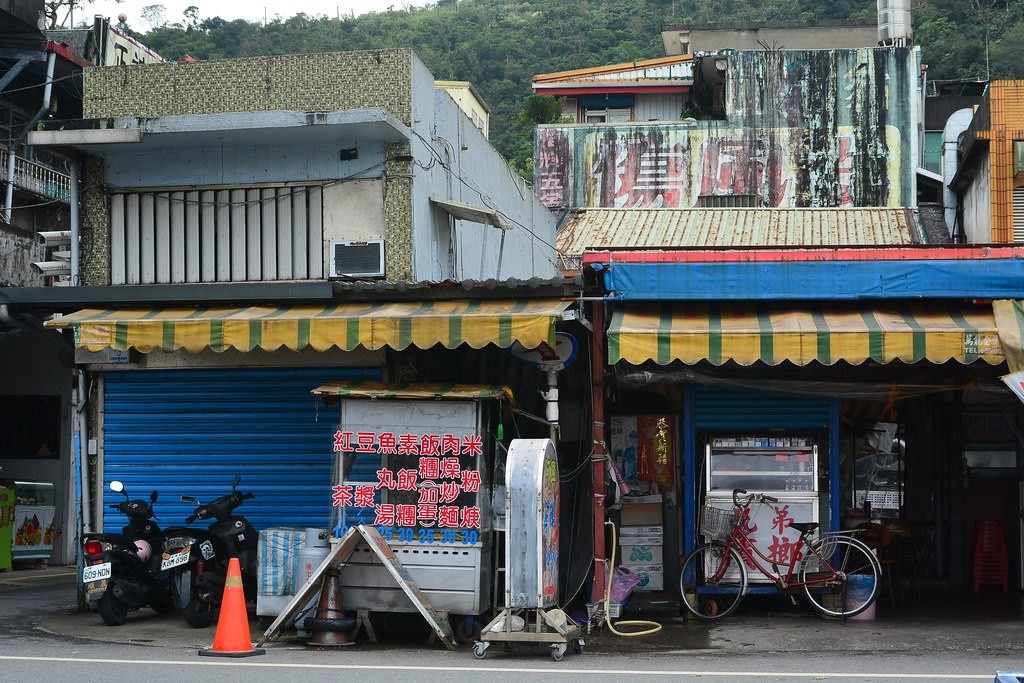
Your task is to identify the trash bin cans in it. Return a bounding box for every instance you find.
[847,573,880,622]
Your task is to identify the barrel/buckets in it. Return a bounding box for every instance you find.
[846,575,878,621]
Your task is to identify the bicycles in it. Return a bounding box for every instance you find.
[678,490,884,625]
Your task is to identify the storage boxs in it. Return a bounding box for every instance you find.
[619,526,663,536]
[621,503,663,527]
[619,535,663,546]
[622,494,663,505]
[621,546,663,565]
[619,564,664,591]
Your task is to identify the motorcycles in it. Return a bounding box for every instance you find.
[159,475,263,628]
[80,479,176,627]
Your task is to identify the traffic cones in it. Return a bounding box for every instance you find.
[197,558,266,658]
[309,570,355,647]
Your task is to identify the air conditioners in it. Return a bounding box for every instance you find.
[330,240,386,278]
